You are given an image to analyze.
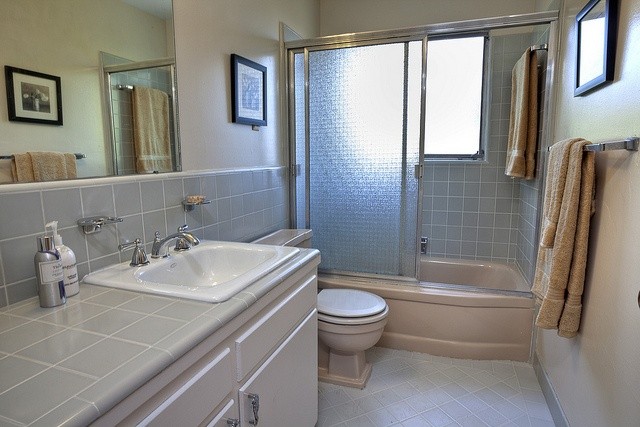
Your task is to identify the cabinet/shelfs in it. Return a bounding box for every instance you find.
[91,319,232,426]
[232,255,322,425]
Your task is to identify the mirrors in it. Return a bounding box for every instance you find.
[0,0,183,185]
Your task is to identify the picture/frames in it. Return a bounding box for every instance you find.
[228,53,269,128]
[571,0,619,96]
[3,65,64,128]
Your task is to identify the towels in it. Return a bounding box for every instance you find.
[9,150,78,180]
[502,44,545,182]
[128,84,175,175]
[528,136,598,340]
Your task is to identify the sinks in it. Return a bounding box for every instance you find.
[84,238,299,304]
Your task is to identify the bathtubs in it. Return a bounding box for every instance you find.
[317,255,534,363]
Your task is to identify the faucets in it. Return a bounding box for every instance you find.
[152,230,200,258]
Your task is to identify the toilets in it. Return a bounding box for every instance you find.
[251,228,390,390]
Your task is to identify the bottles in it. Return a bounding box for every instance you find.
[33,232,66,308]
